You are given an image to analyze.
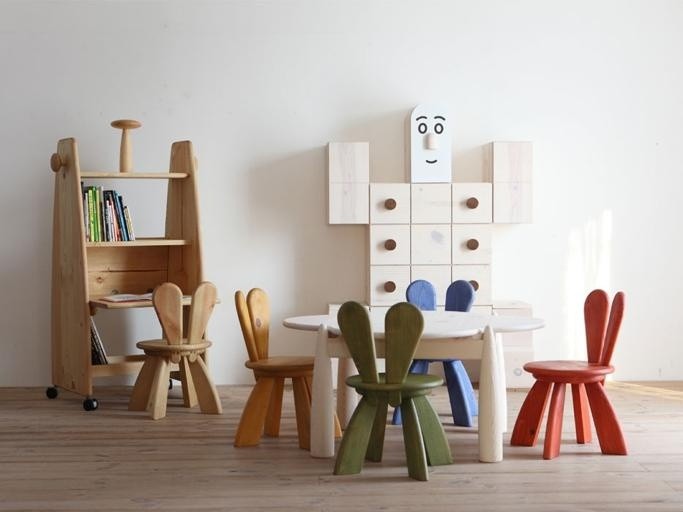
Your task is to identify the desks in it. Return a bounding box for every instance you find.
[282,311,545,463]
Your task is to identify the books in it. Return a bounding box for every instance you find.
[102,292,152,302]
[82,181,135,242]
[89,315,110,366]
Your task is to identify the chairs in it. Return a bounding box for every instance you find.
[391,280,479,428]
[234,286,343,450]
[333,301,452,482]
[508,289,628,460]
[128,282,224,419]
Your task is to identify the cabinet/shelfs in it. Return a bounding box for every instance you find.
[325,101,545,391]
[45,119,204,411]
